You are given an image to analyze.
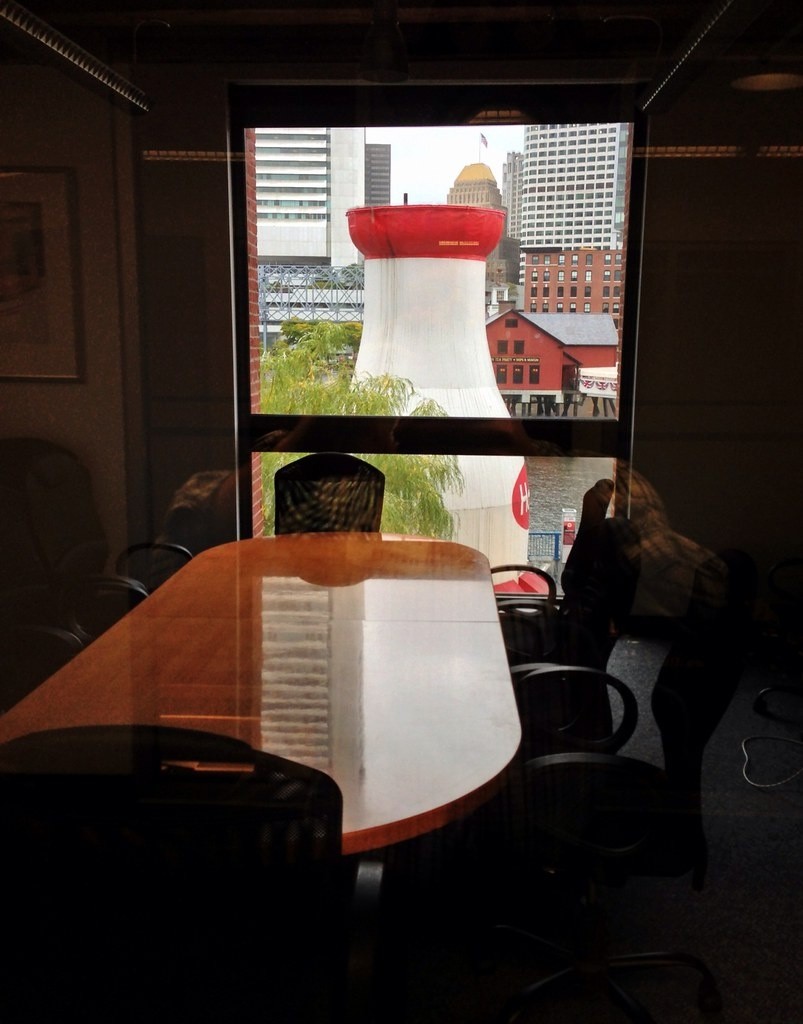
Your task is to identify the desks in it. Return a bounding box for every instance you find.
[1,532,526,855]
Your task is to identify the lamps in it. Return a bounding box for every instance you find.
[731,63,803,91]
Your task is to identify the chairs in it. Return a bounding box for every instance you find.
[1,437,802,1024]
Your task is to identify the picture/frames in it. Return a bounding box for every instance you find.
[0,165,87,384]
